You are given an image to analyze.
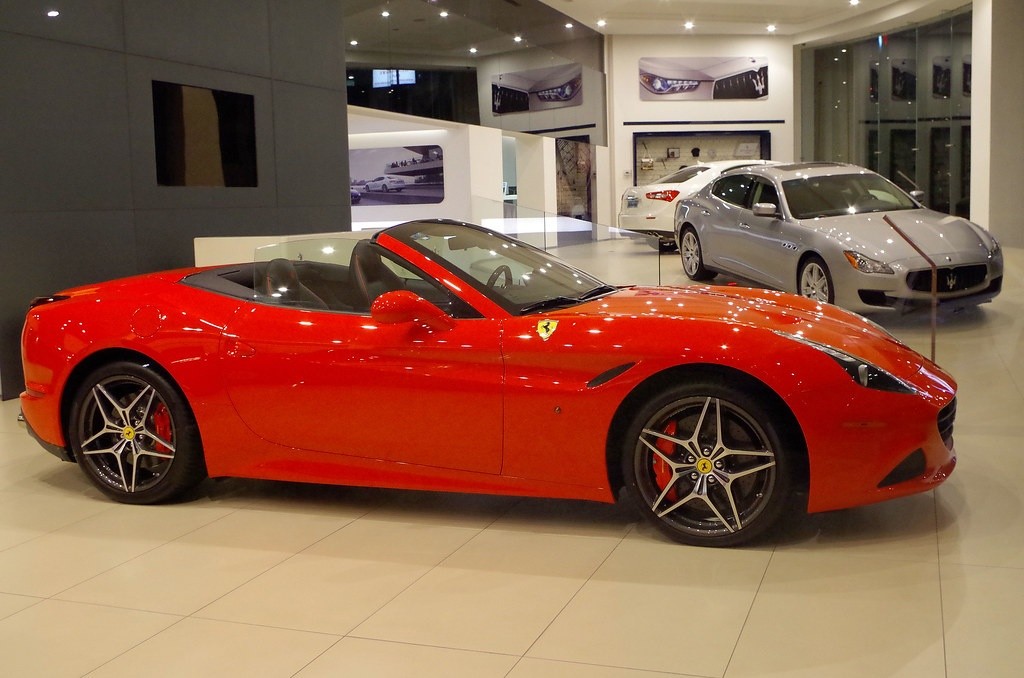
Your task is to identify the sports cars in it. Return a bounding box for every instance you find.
[21,220,959,548]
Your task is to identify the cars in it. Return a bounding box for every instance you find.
[617,160,904,252]
[673,160,1005,314]
[350,188,361,203]
[366,176,406,192]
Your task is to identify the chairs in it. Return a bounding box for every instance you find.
[262,258,329,310]
[345,239,407,311]
